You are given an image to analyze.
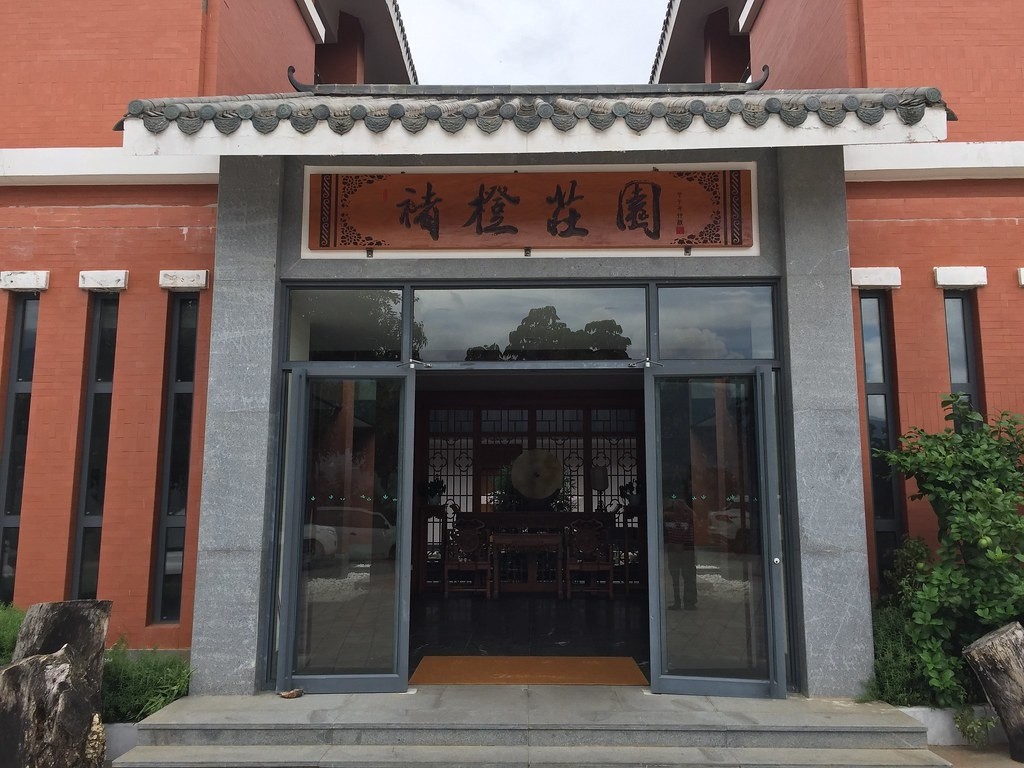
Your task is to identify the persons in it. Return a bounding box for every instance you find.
[664,499,698,611]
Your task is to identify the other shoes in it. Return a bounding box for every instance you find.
[684,603,696,609]
[668,600,680,608]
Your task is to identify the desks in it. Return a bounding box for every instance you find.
[454,512,615,595]
[623,504,646,596]
[419,504,447,592]
[492,532,564,600]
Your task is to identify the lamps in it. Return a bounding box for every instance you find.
[590,466,608,512]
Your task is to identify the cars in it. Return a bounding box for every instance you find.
[303,523,338,570]
[707,502,751,551]
[308,507,396,560]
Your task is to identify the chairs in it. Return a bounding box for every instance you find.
[445,518,491,599]
[565,518,615,600]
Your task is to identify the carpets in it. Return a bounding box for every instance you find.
[411,656,650,686]
